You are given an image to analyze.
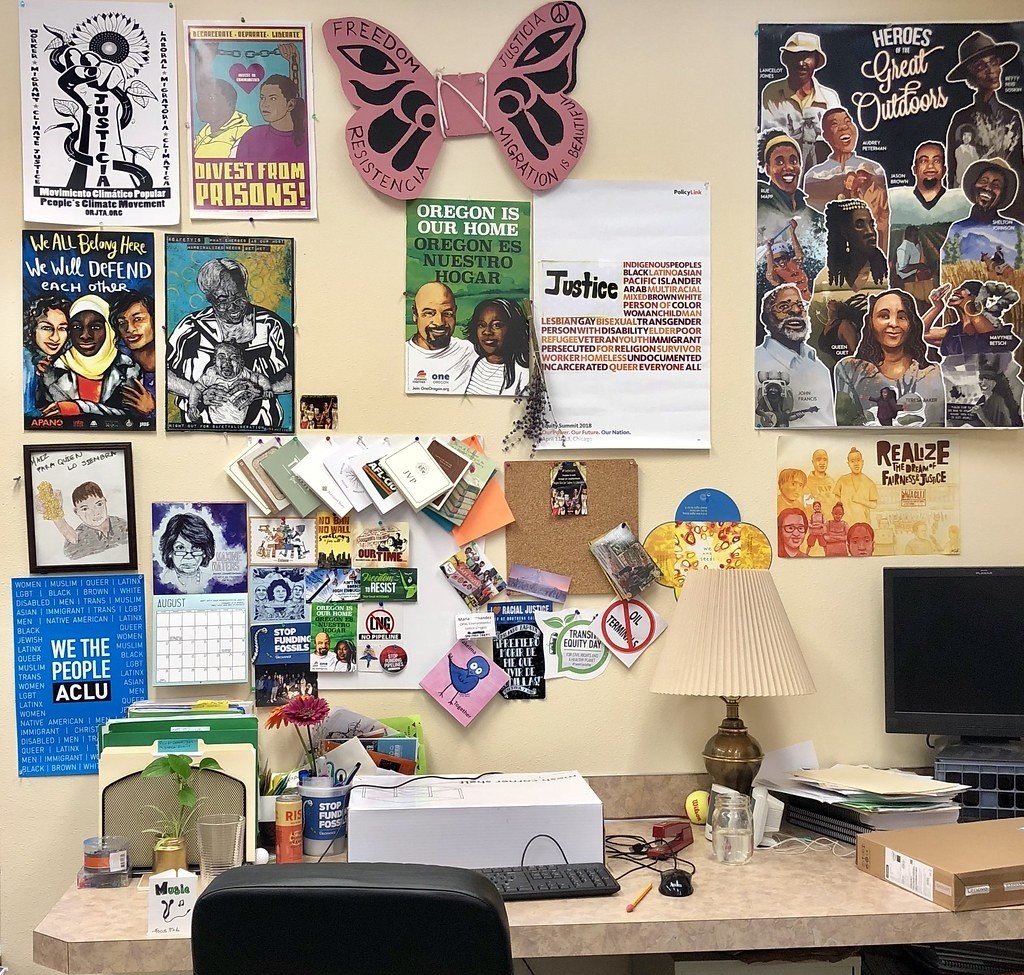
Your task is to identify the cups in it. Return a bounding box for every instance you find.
[196,814,245,888]
[297,781,352,856]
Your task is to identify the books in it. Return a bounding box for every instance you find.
[912,942,1024,975]
[772,774,962,846]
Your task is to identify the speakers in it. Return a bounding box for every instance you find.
[750,787,785,849]
[705,784,740,841]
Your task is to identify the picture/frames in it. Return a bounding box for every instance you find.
[23,442,138,573]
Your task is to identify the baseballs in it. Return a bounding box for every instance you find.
[684,790,711,826]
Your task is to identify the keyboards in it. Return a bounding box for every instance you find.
[470,862,620,902]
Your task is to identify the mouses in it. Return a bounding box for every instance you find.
[659,869,694,897]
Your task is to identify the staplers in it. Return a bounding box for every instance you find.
[646,820,694,859]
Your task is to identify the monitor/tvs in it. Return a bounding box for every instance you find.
[884,566,1024,763]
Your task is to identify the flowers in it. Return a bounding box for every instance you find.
[266,695,330,775]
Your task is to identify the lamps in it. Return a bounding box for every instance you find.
[648,568,817,797]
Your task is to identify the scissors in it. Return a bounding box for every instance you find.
[326,761,347,787]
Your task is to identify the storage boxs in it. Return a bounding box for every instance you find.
[346,771,606,870]
[855,818,1024,914]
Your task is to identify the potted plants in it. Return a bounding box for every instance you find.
[137,752,225,876]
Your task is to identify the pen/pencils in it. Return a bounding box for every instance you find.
[308,768,313,778]
[339,774,343,781]
[627,883,653,912]
[343,762,362,786]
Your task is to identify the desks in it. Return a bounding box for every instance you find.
[33,814,1024,975]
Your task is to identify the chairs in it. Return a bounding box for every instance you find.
[190,863,513,975]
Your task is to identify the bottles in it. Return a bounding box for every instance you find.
[712,793,754,865]
[276,795,302,863]
